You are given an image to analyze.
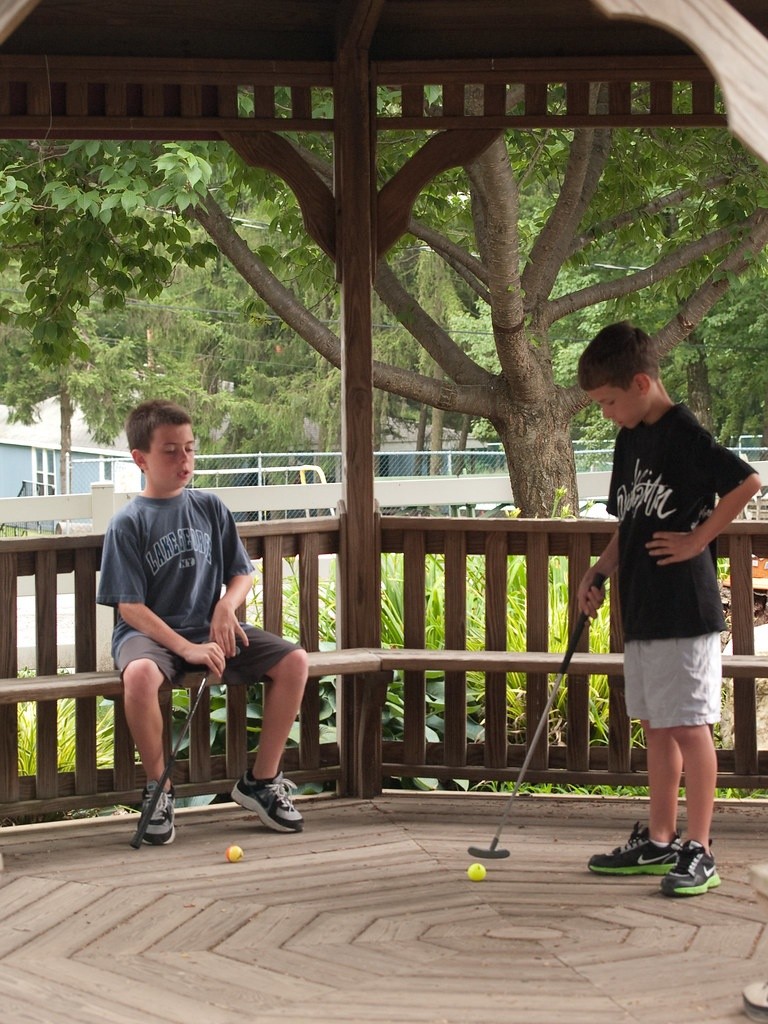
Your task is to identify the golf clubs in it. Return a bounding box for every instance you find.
[128,640,242,851]
[464,571,608,861]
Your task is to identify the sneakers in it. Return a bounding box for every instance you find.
[588,820,681,875]
[660,838,721,895]
[232,767,304,832]
[137,780,175,845]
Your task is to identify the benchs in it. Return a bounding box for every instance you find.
[0,647,768,821]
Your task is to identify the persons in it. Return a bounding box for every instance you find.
[577,321,760,893]
[96,400,309,846]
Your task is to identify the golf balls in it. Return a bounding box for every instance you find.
[226,845,244,863]
[468,862,487,881]
